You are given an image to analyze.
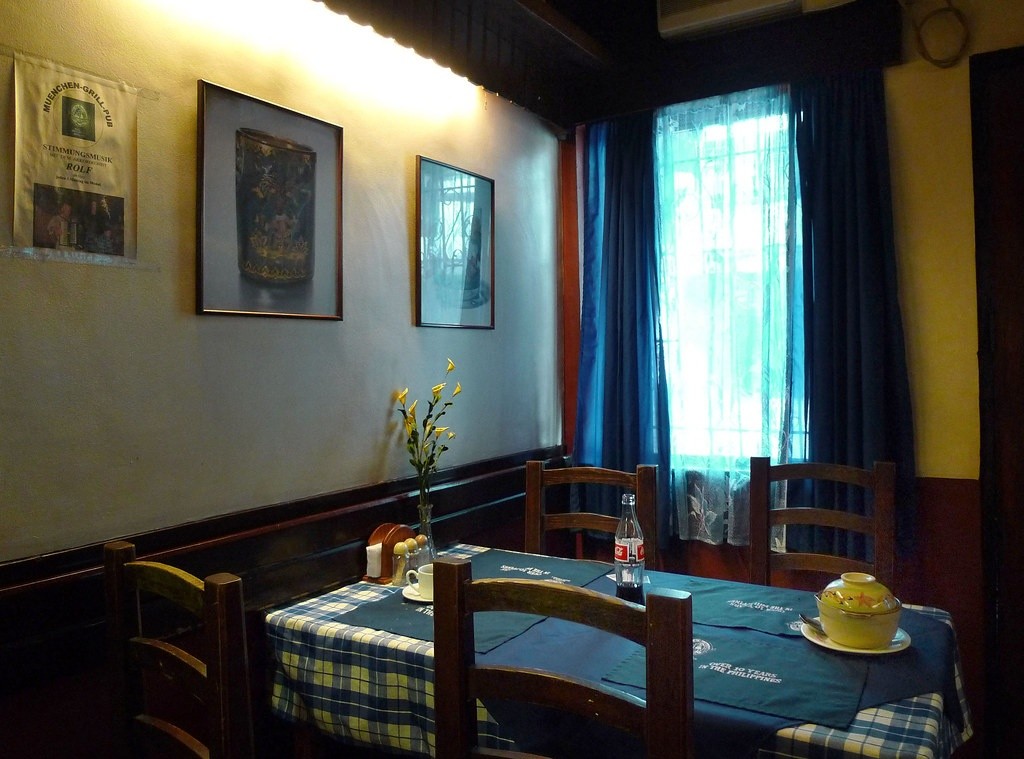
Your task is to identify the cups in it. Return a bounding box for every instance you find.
[406,564,434,599]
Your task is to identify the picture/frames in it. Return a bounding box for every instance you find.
[191,78,344,322]
[412,152,497,330]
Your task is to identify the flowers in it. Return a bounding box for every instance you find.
[398,357,463,505]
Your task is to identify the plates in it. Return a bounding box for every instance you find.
[401,581,433,603]
[801,617,911,656]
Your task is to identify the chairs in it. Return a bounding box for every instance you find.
[749,456,894,593]
[433,557,693,758]
[103,540,255,759]
[527,460,658,567]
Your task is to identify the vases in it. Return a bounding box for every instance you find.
[416,507,438,565]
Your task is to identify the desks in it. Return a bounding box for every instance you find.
[263,543,973,759]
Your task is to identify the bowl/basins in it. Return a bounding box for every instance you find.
[814,572,902,650]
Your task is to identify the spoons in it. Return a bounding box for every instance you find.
[799,613,827,636]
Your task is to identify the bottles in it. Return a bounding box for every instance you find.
[392,541,411,587]
[415,534,430,569]
[614,494,646,610]
[403,538,420,579]
